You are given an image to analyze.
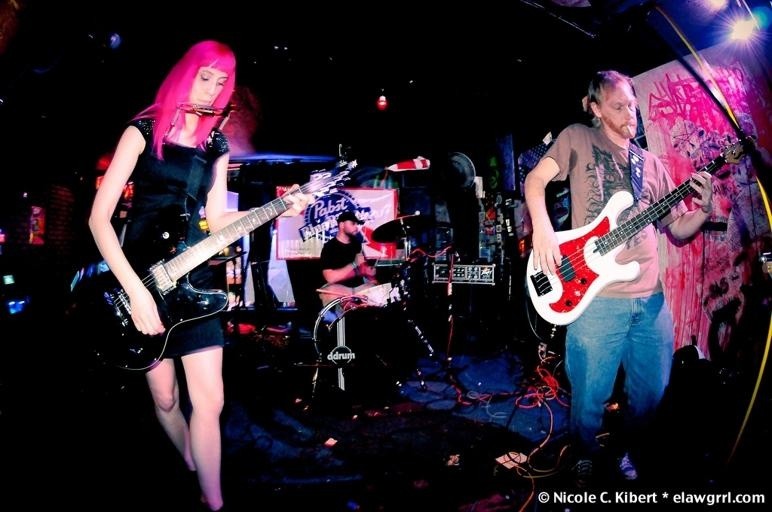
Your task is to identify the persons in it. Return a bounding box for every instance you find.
[88,40,316,512]
[525,70,674,492]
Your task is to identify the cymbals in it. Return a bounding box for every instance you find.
[370,212,433,242]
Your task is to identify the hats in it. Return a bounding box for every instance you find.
[337,212,364,224]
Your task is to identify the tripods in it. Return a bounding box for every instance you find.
[380,228,434,393]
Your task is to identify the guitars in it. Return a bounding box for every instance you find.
[64,158,359,372]
[524,135,758,326]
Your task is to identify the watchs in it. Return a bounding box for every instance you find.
[318,211,377,288]
[352,261,357,269]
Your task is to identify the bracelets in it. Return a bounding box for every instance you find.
[701,206,711,214]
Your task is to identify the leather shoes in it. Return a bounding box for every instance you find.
[617,452,637,480]
[571,459,593,493]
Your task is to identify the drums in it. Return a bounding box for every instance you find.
[312,296,386,370]
